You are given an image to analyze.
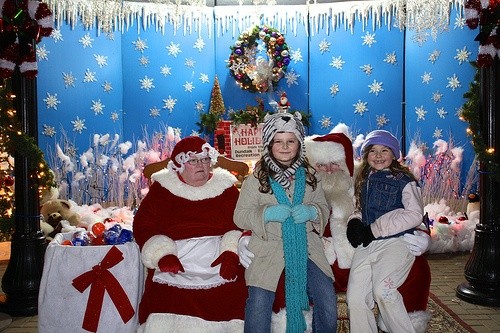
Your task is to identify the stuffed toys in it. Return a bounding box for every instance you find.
[41,199,80,238]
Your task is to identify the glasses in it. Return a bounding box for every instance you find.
[188,158,211,165]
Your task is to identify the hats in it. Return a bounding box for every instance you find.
[303,133,355,178]
[166,136,220,175]
[360,130,400,159]
[261,111,306,188]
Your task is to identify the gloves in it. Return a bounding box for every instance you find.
[264,204,292,223]
[291,204,318,224]
[158,254,184,274]
[238,235,254,270]
[403,230,433,256]
[211,250,240,280]
[346,218,376,248]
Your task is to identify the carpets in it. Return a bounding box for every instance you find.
[334,294,478,333]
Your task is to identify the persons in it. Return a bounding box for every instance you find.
[233,112,432,333]
[133,136,249,333]
